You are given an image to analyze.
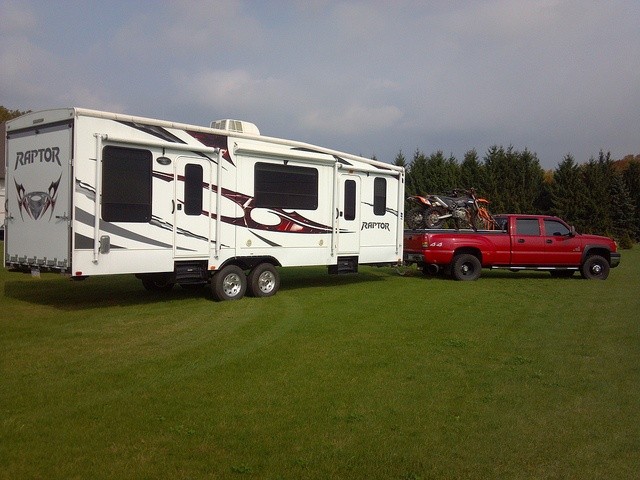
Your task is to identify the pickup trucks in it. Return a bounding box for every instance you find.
[404,214,621,281]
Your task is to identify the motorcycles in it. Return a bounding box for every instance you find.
[424,187,491,229]
[404,190,461,229]
